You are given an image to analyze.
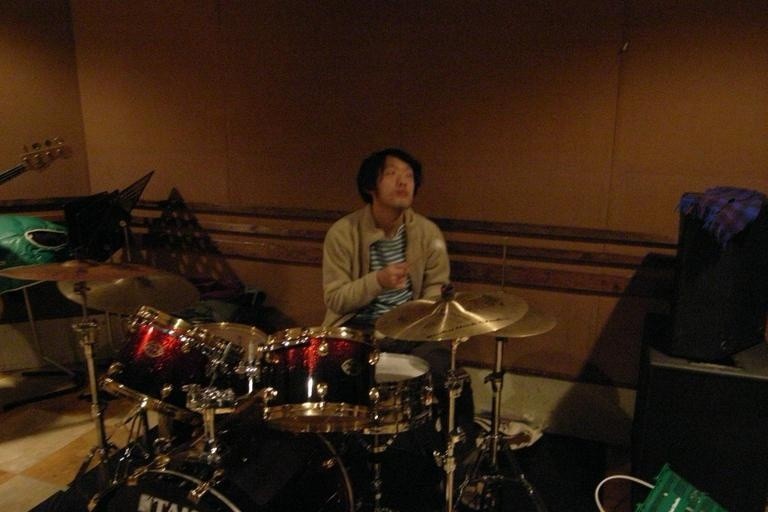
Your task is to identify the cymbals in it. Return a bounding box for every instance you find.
[0,260,153,282]
[481,307,558,337]
[56,264,200,315]
[375,287,529,343]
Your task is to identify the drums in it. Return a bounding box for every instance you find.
[85,424,357,512]
[188,322,271,416]
[373,352,433,436]
[95,304,245,426]
[257,326,379,431]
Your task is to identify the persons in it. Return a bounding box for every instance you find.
[320,147,475,424]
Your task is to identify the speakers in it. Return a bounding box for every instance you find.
[667,185,768,360]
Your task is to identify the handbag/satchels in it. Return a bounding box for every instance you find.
[595,464,726,512]
